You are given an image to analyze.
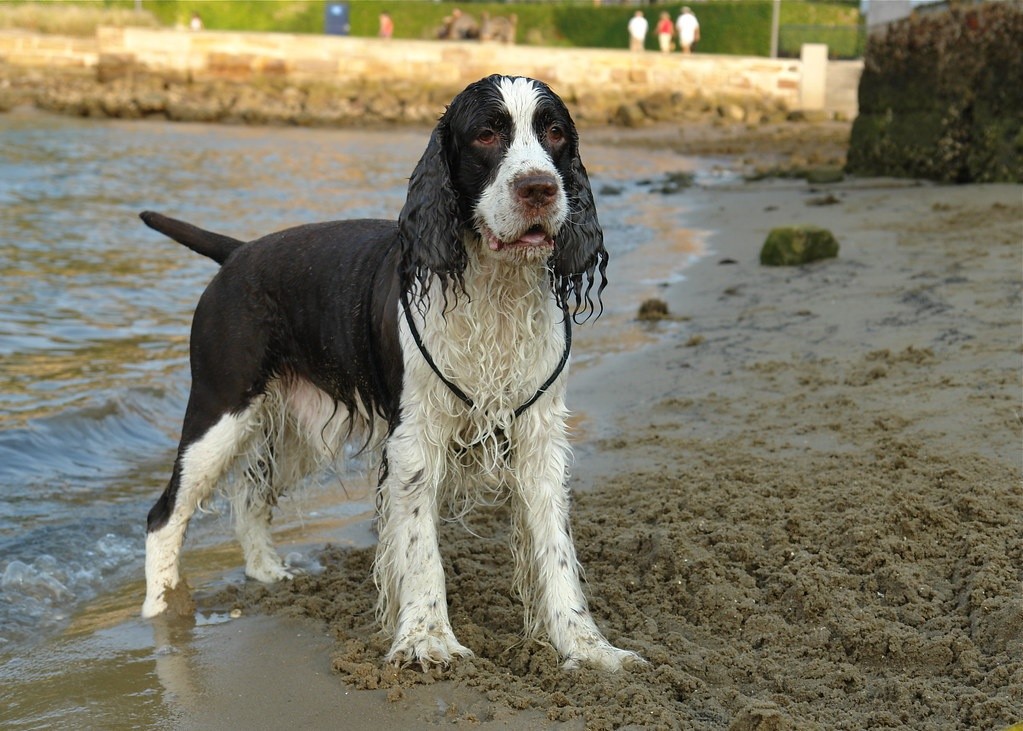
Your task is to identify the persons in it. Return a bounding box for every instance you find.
[627,9,649,51]
[435,8,519,43]
[675,6,700,53]
[654,10,674,53]
[377,9,394,39]
[190,11,206,32]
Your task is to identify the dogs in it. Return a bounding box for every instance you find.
[139,72,655,678]
